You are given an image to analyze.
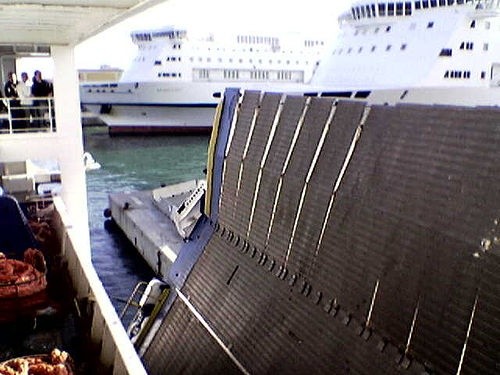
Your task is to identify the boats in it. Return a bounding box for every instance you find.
[81,25,331,140]
[273,0,500,109]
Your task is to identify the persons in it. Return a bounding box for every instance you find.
[31,70,53,132]
[4,70,27,133]
[31,75,37,83]
[15,72,34,131]
